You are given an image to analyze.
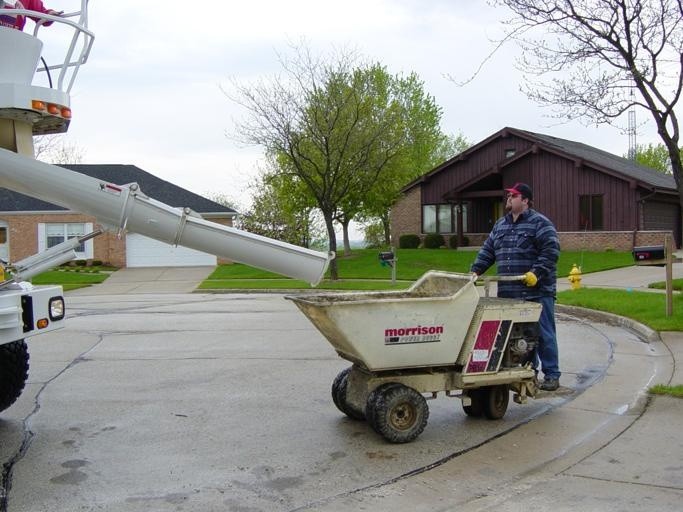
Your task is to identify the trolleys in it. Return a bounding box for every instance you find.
[283,269,543,443]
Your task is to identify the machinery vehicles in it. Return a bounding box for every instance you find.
[1,0,330,425]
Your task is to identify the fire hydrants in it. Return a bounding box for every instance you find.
[568,264,581,290]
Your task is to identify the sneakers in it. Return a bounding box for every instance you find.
[539,378,559,391]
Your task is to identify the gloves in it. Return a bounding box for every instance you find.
[524,272,538,286]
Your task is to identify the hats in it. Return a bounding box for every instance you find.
[504,183,532,198]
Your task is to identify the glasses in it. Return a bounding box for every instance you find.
[506,193,518,198]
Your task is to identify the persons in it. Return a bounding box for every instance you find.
[469,182,562,391]
